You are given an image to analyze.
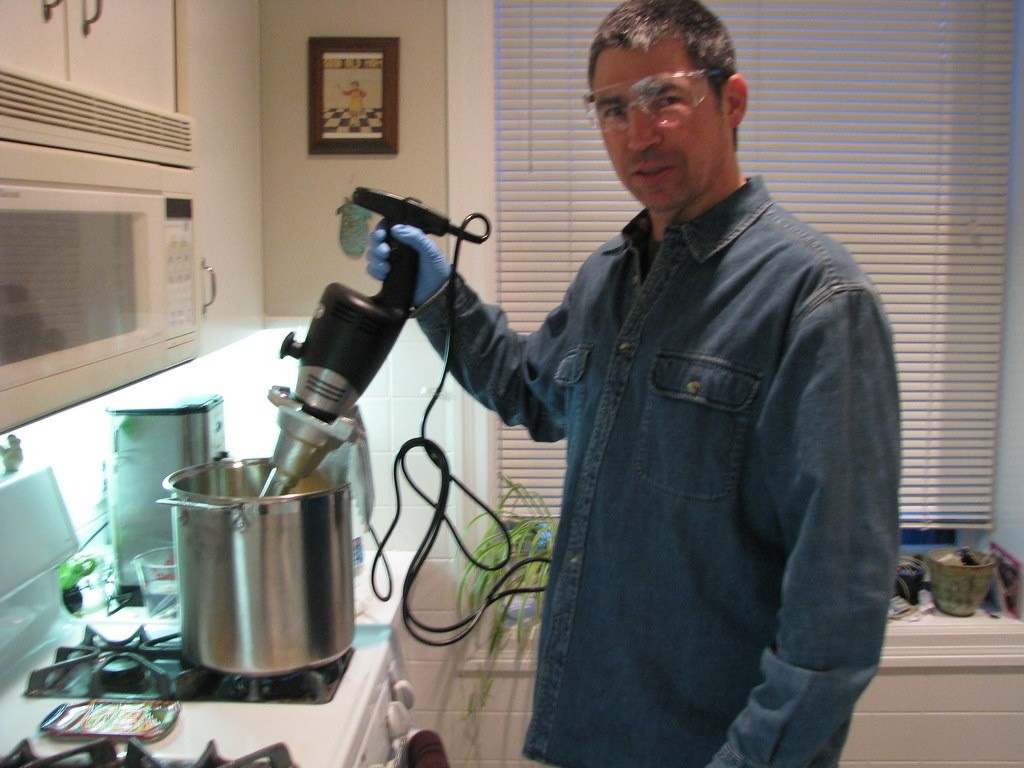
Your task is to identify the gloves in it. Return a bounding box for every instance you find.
[366,217,450,310]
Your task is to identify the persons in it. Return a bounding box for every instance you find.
[365,1,902,767]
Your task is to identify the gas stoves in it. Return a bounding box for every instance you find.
[0,467,418,768]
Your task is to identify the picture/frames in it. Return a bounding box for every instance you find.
[306,35,401,155]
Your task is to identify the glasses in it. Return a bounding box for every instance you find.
[583,67,731,133]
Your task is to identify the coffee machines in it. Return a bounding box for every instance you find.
[107,392,225,612]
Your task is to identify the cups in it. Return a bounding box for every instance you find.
[926,548,998,617]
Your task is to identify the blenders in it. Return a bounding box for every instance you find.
[251,188,454,499]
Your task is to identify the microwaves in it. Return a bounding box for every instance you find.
[1,179,204,438]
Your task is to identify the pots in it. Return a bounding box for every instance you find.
[153,457,354,677]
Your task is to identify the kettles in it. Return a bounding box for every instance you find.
[314,406,376,575]
